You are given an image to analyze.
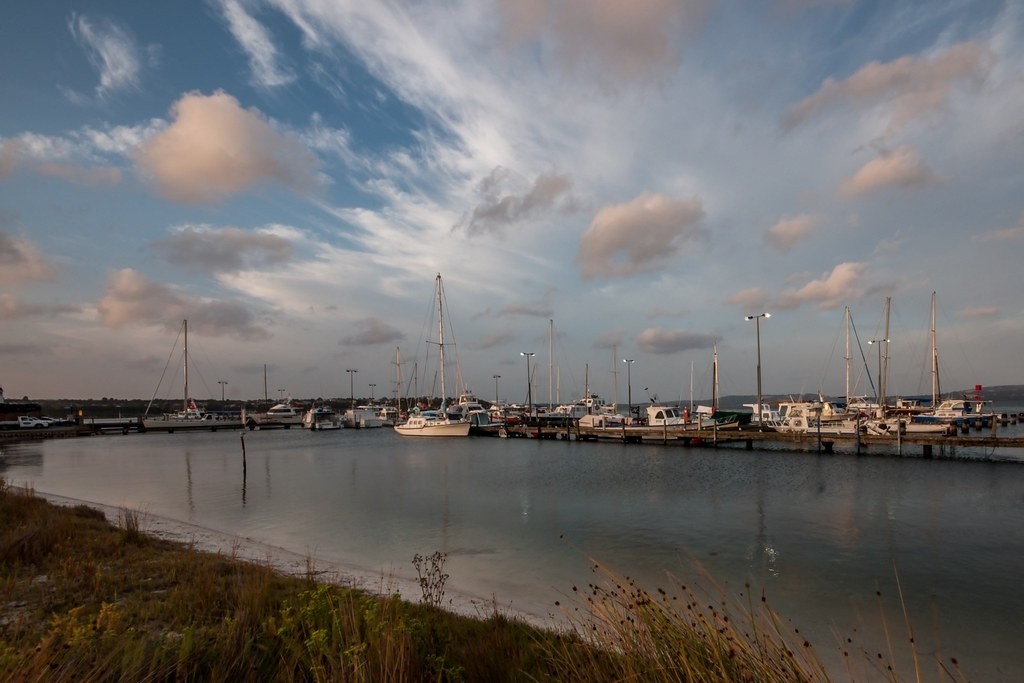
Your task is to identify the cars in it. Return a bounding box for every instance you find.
[41,415,72,427]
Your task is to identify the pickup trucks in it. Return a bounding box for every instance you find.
[0,415,49,429]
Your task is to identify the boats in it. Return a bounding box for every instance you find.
[302,396,344,431]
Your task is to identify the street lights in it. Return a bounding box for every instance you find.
[369,384,378,402]
[346,369,357,402]
[519,352,536,422]
[492,374,502,408]
[622,359,635,419]
[217,380,228,402]
[868,335,891,403]
[275,388,286,403]
[745,311,772,428]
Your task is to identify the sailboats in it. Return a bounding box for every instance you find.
[392,271,474,437]
[345,290,1007,428]
[131,318,245,431]
[245,363,302,425]
